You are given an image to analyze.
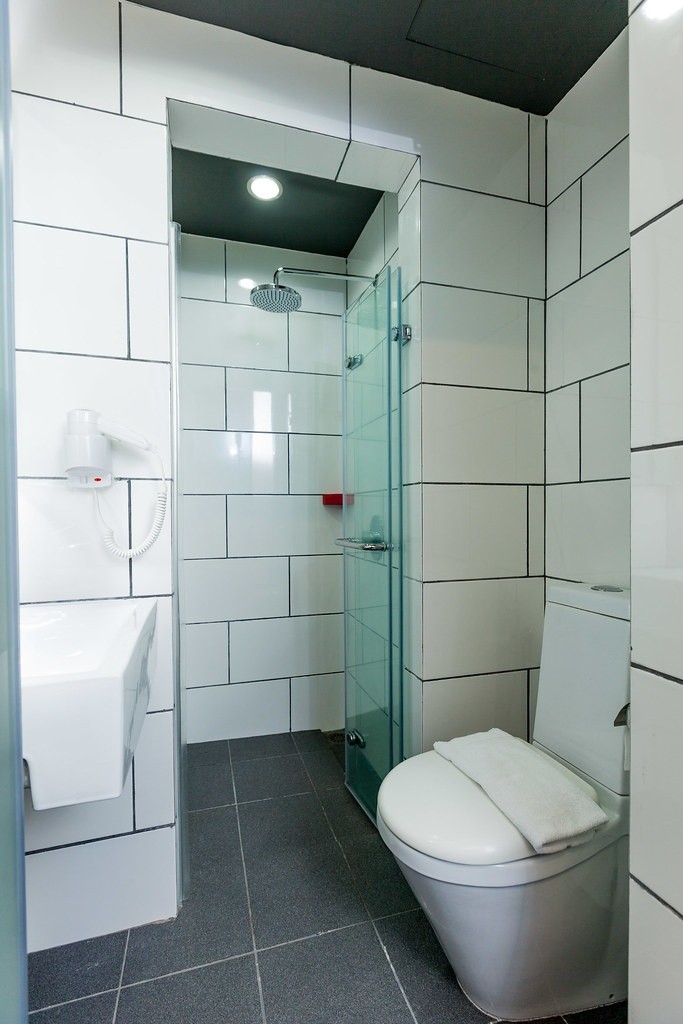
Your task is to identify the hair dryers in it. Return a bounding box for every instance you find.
[63,409,155,479]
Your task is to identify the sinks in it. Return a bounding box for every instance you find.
[20,596,158,811]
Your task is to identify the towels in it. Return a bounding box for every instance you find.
[433,727,610,855]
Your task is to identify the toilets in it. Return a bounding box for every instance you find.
[372,566,628,1024]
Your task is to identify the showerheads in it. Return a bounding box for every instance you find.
[250,282,301,314]
